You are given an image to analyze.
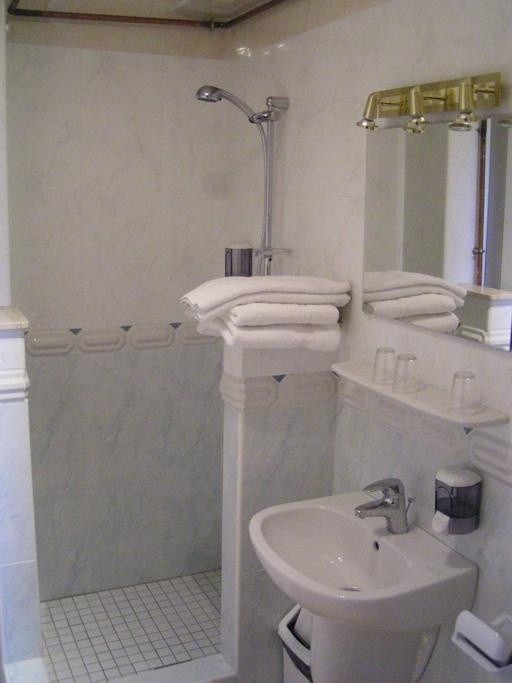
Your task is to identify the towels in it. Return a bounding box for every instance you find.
[362,269,468,334]
[182,275,351,356]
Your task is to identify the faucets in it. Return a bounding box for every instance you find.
[355,478,414,534]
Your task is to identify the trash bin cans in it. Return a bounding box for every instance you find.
[277,602,313,683]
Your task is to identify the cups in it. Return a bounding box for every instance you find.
[372,344,396,392]
[445,370,487,416]
[393,354,417,394]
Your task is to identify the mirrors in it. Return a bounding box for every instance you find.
[359,115,511,349]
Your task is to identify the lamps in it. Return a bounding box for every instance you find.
[357,71,503,134]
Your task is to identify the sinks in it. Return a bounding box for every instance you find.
[250,490,480,632]
